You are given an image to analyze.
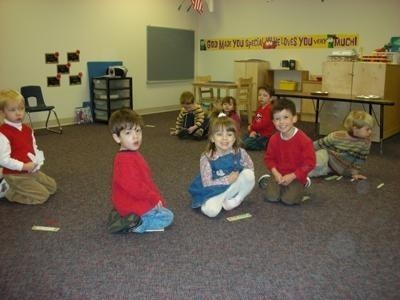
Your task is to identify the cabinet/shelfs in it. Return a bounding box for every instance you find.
[193,80,253,126]
[92,77,133,125]
[233,59,269,115]
[320,60,400,143]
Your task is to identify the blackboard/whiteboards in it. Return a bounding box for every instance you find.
[146,25,197,86]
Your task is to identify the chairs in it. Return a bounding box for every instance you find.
[21,86,62,135]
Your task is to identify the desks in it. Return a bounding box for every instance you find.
[270,87,395,156]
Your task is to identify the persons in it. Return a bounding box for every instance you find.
[240,84,276,150]
[211,96,242,138]
[258,98,318,206]
[188,109,257,218]
[0,89,57,205]
[173,91,211,141]
[104,109,173,235]
[307,110,374,186]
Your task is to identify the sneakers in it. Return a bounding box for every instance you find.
[107,213,142,234]
[0,177,9,198]
[304,176,312,191]
[258,174,271,190]
[107,209,120,226]
